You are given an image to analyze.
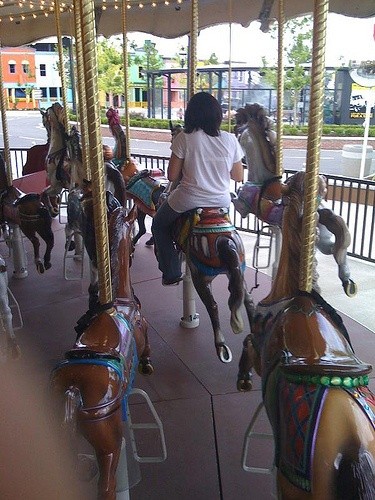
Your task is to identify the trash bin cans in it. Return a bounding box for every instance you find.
[341,144,373,180]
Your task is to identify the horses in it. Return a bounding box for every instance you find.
[0,102,375,500]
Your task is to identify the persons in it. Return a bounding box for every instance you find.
[150,91,245,287]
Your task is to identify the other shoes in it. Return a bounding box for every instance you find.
[162,273,184,284]
